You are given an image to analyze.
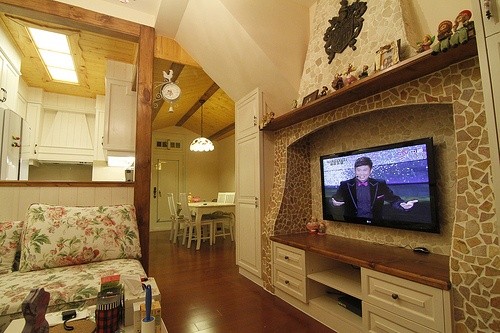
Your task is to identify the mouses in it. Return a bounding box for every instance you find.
[412,246,430,254]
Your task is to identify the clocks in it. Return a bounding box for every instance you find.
[161,70,181,112]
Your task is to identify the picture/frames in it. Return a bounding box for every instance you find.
[302,89,319,106]
[375,39,401,73]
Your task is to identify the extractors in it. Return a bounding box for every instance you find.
[36,111,94,165]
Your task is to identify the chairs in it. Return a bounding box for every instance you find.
[167,191,235,250]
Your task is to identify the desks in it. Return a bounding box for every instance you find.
[176,202,236,250]
[4,298,169,333]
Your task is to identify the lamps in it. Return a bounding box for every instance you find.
[189,105,215,153]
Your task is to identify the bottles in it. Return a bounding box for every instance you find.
[187,192,191,203]
[306,217,325,234]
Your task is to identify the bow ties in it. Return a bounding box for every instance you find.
[358,182,368,187]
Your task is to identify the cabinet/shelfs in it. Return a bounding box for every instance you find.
[235,88,452,333]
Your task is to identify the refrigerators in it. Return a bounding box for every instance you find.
[0,107,32,181]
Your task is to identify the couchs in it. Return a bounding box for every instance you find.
[0,203,148,333]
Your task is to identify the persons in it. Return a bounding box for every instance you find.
[328,157,419,220]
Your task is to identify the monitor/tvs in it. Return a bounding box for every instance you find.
[319,136,441,235]
